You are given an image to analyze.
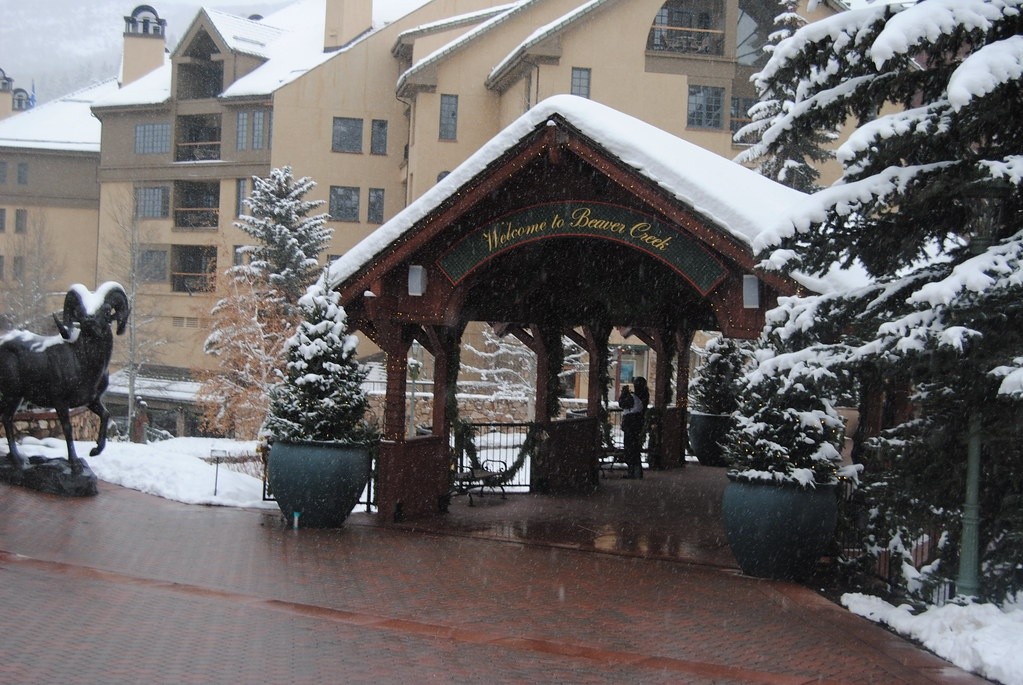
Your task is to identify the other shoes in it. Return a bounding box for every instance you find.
[621,469,643,480]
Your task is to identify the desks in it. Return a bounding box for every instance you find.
[673,36,697,53]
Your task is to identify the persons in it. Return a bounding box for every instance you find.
[128,395,151,444]
[880,364,936,471]
[618,377,649,479]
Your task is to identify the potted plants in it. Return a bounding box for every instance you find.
[688,333,747,466]
[264,255,384,527]
[716,322,849,581]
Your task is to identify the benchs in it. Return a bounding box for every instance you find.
[450,460,507,508]
[600,442,627,478]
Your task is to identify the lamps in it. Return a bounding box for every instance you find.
[407,265,427,296]
[743,274,761,308]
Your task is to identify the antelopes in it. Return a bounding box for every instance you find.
[0,280,132,466]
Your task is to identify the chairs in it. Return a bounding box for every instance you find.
[658,34,711,54]
